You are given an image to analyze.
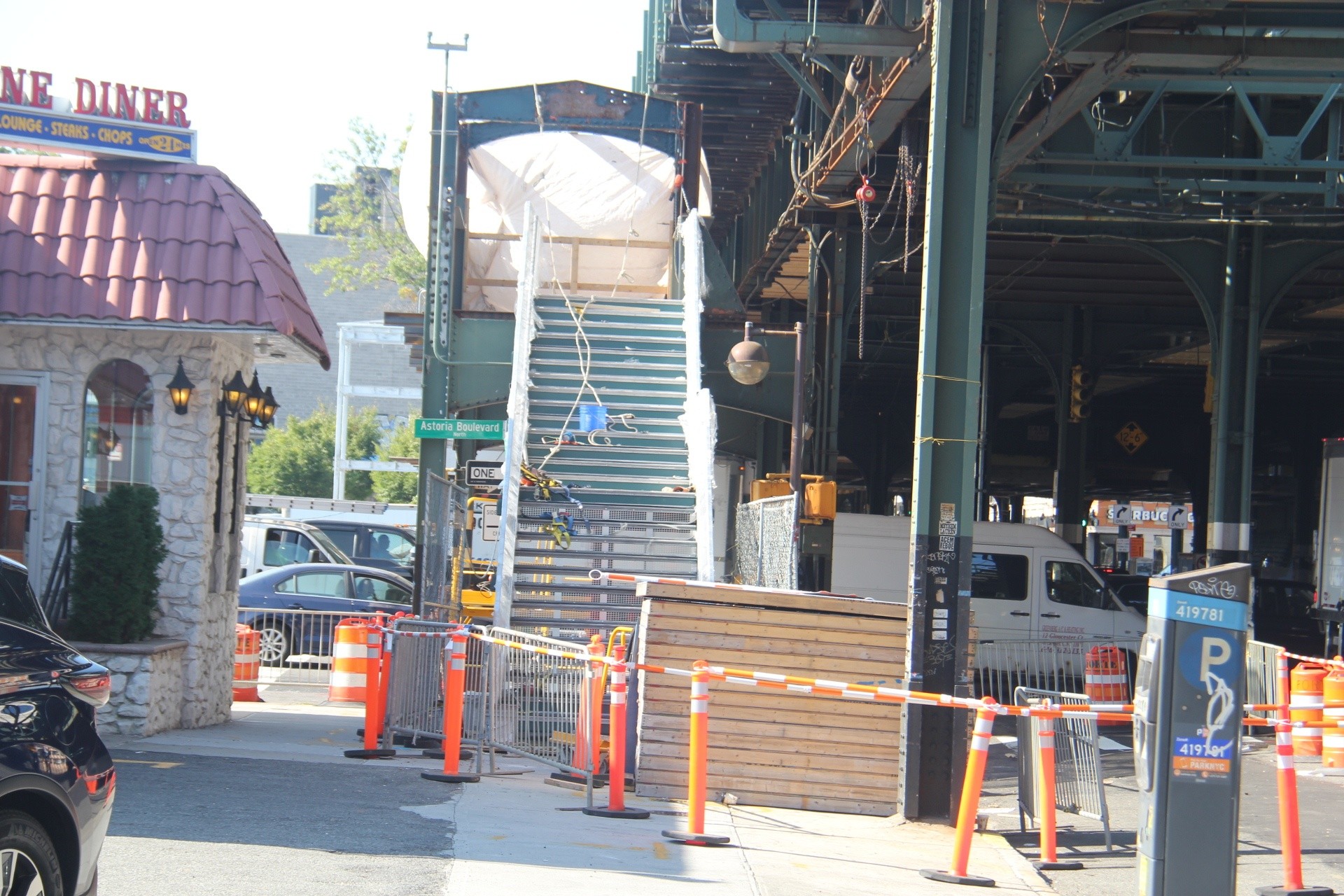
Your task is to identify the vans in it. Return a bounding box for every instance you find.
[241,514,357,596]
[831,511,1148,706]
[278,518,417,583]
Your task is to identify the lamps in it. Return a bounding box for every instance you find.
[251,386,281,429]
[254,343,273,354]
[238,368,268,422]
[87,425,121,456]
[216,370,251,417]
[165,355,196,415]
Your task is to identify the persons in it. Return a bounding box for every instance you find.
[356,579,374,600]
[378,535,392,559]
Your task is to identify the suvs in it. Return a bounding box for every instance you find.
[0,556,117,896]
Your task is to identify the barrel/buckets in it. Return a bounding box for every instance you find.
[578,405,606,432]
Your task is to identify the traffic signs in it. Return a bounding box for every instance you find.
[1168,505,1187,530]
[1113,504,1131,526]
[466,460,506,486]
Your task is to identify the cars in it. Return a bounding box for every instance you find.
[1091,564,1152,619]
[237,563,493,667]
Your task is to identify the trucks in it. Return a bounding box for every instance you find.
[1308,437,1343,658]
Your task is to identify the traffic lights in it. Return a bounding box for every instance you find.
[1069,365,1093,424]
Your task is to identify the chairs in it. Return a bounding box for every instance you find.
[335,579,345,598]
[370,537,378,557]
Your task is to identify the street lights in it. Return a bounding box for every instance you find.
[725,320,805,493]
